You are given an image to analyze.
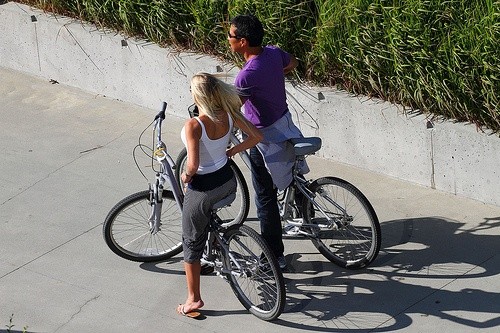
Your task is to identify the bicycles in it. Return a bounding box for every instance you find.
[175,65,382,269]
[104,100,286,323]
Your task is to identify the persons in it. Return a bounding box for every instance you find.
[225,15,318,238]
[174,72,262,318]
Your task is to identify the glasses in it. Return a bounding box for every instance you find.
[228,30,239,38]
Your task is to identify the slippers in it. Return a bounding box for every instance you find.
[177,304,201,318]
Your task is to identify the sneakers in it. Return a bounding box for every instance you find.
[258,255,288,271]
[284,219,320,238]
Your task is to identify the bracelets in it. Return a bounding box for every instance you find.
[185,172,189,177]
